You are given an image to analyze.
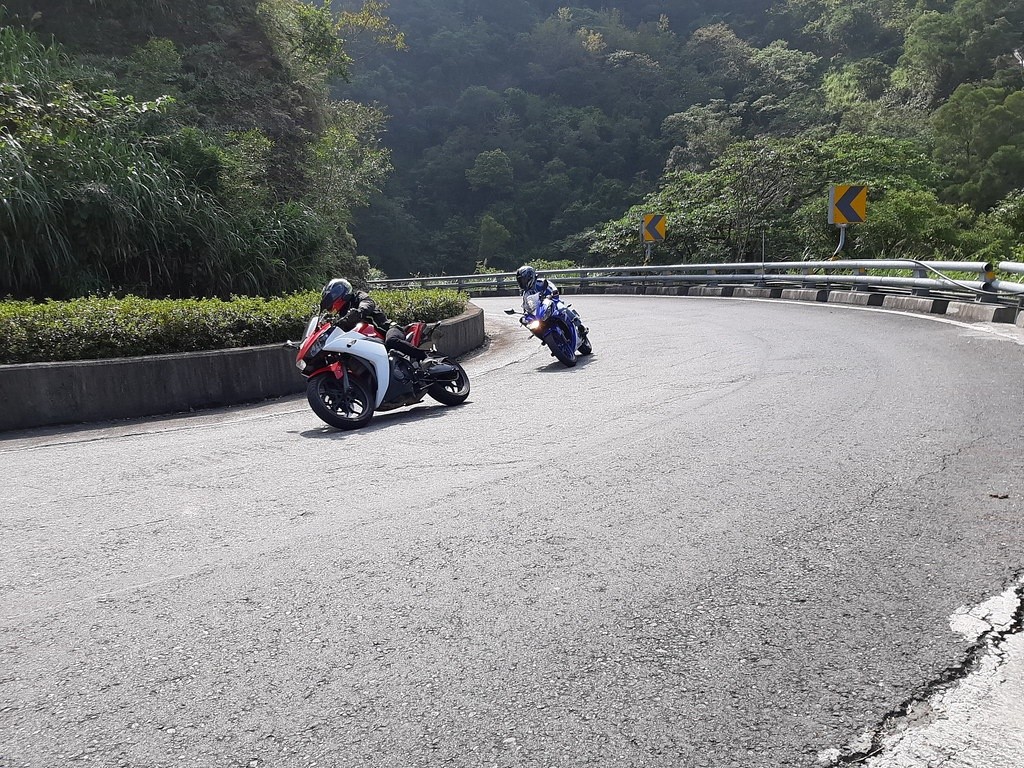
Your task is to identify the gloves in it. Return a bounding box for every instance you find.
[347,307,362,322]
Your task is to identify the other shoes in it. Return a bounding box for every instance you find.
[578,325,587,334]
[421,357,438,368]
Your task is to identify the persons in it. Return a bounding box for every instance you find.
[318,278,434,367]
[514,265,588,339]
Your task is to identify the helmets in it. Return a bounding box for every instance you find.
[516,266,536,292]
[320,278,353,314]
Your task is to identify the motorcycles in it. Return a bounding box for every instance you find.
[284,308,470,430]
[503,279,592,366]
